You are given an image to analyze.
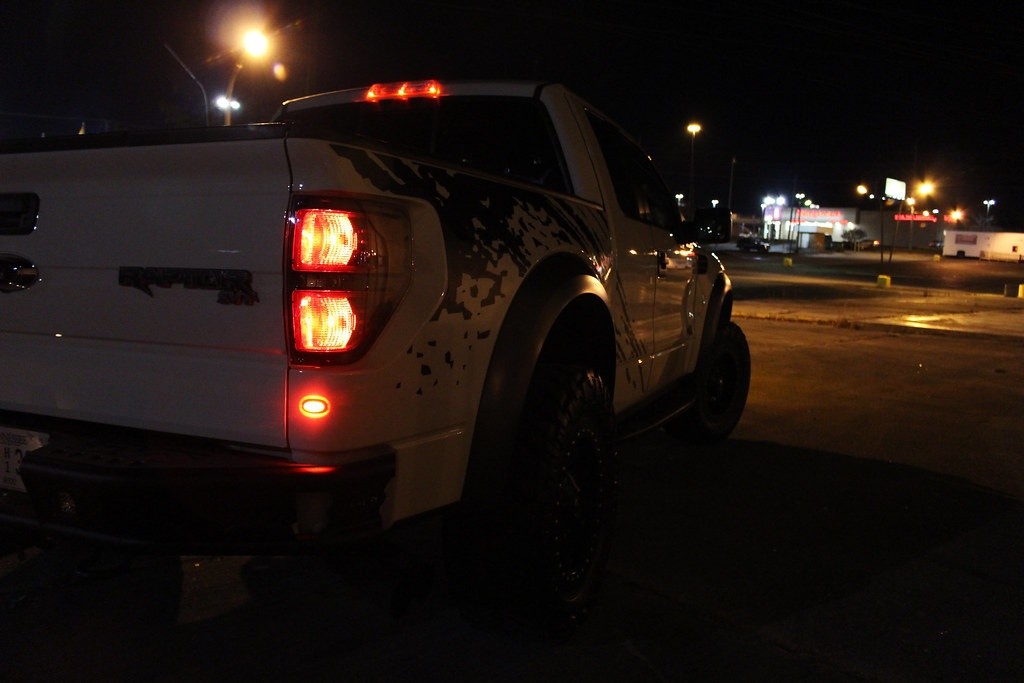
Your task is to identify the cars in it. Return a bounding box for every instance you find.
[735,237,771,254]
[928,240,943,248]
[853,239,878,251]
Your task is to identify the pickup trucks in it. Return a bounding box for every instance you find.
[0,80,753,596]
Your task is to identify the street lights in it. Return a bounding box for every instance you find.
[222,9,273,126]
[983,199,995,230]
[910,180,933,252]
[687,121,699,204]
[763,193,805,254]
